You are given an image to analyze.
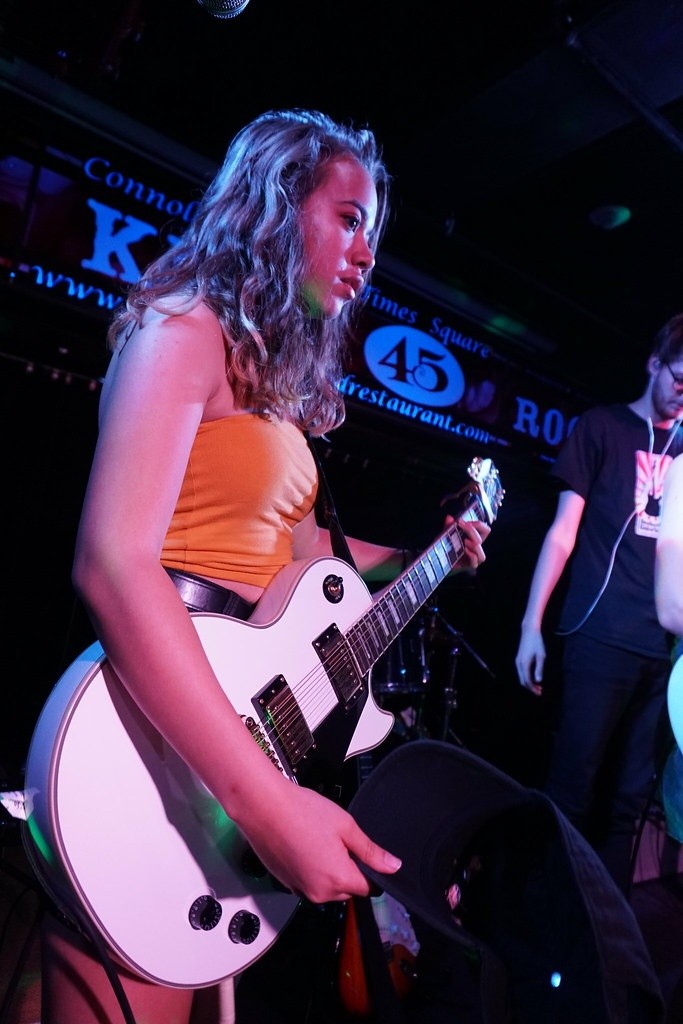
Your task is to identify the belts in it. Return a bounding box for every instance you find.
[164,569,255,619]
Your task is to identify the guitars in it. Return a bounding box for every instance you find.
[23,456,510,987]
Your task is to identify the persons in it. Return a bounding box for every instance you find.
[19,106,492,1024]
[516,320,683,874]
[654,454,683,642]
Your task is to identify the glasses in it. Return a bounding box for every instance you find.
[665,362,683,391]
